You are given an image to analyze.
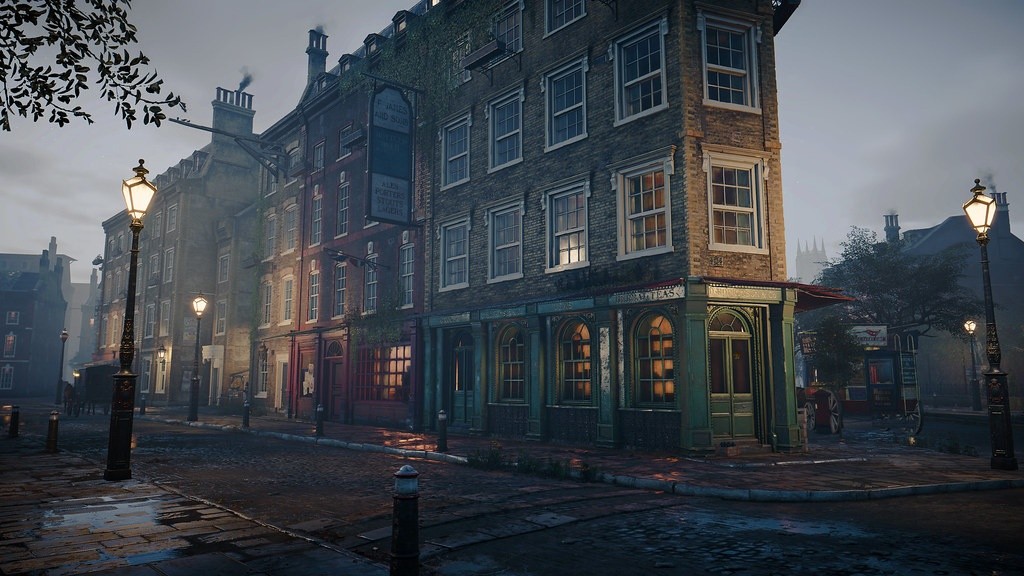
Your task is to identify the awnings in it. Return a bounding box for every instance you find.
[794,283,855,314]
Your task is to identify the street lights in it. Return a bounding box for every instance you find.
[104,157,157,480]
[962,179,1017,471]
[55,330,68,408]
[964,318,983,411]
[189,290,208,422]
